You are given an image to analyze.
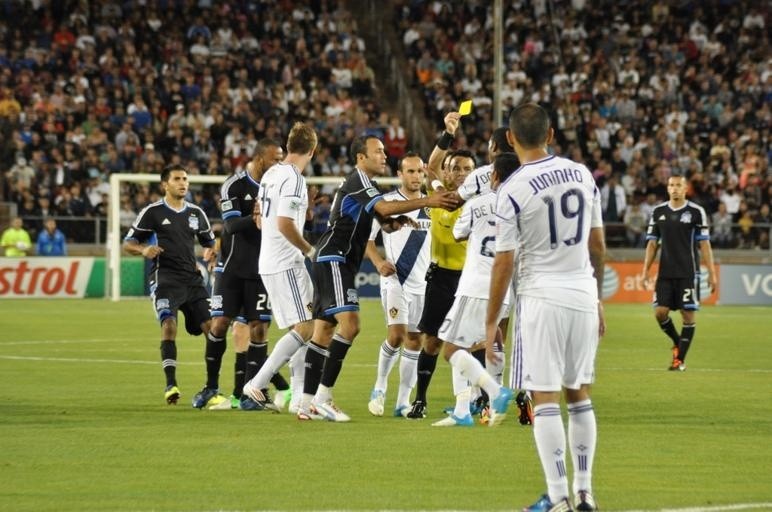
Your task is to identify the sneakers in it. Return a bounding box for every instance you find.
[242,379,281,414]
[208,394,227,404]
[480,406,490,424]
[672,347,679,361]
[368,383,385,417]
[289,398,309,413]
[164,385,181,404]
[431,414,474,426]
[488,387,513,427]
[241,395,264,411]
[209,395,240,410]
[393,404,413,417]
[297,407,324,420]
[192,385,220,410]
[274,385,291,409]
[516,391,534,425]
[574,490,598,512]
[523,494,574,512]
[310,401,351,422]
[470,400,489,416]
[668,359,687,372]
[407,400,426,418]
[443,408,454,415]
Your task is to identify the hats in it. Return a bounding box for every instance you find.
[434,78,443,85]
[176,104,184,111]
[17,157,26,166]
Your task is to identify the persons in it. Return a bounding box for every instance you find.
[192,139,282,412]
[420,127,534,426]
[641,174,716,373]
[1,163,162,257]
[426,112,452,188]
[407,150,491,418]
[364,151,431,417]
[239,121,316,414]
[207,315,289,411]
[393,0,606,159]
[432,154,519,426]
[606,2,772,235]
[298,134,387,421]
[1,1,406,163]
[485,104,607,512]
[122,164,214,402]
